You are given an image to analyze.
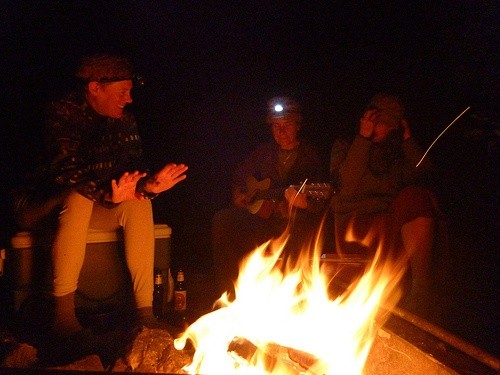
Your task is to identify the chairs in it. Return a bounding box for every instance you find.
[327,137,447,287]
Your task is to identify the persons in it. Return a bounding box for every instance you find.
[21,39,189,350]
[328,92,443,303]
[211,95,334,290]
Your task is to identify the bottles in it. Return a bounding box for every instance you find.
[172,268,188,328]
[152,269,163,321]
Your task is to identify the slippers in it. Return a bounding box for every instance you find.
[50,325,105,361]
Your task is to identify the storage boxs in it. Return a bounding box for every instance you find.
[11,224,172,313]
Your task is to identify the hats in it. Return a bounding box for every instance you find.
[369,98,403,128]
[266,97,301,125]
[74,51,132,82]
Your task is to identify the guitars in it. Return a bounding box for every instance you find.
[236,175,333,219]
